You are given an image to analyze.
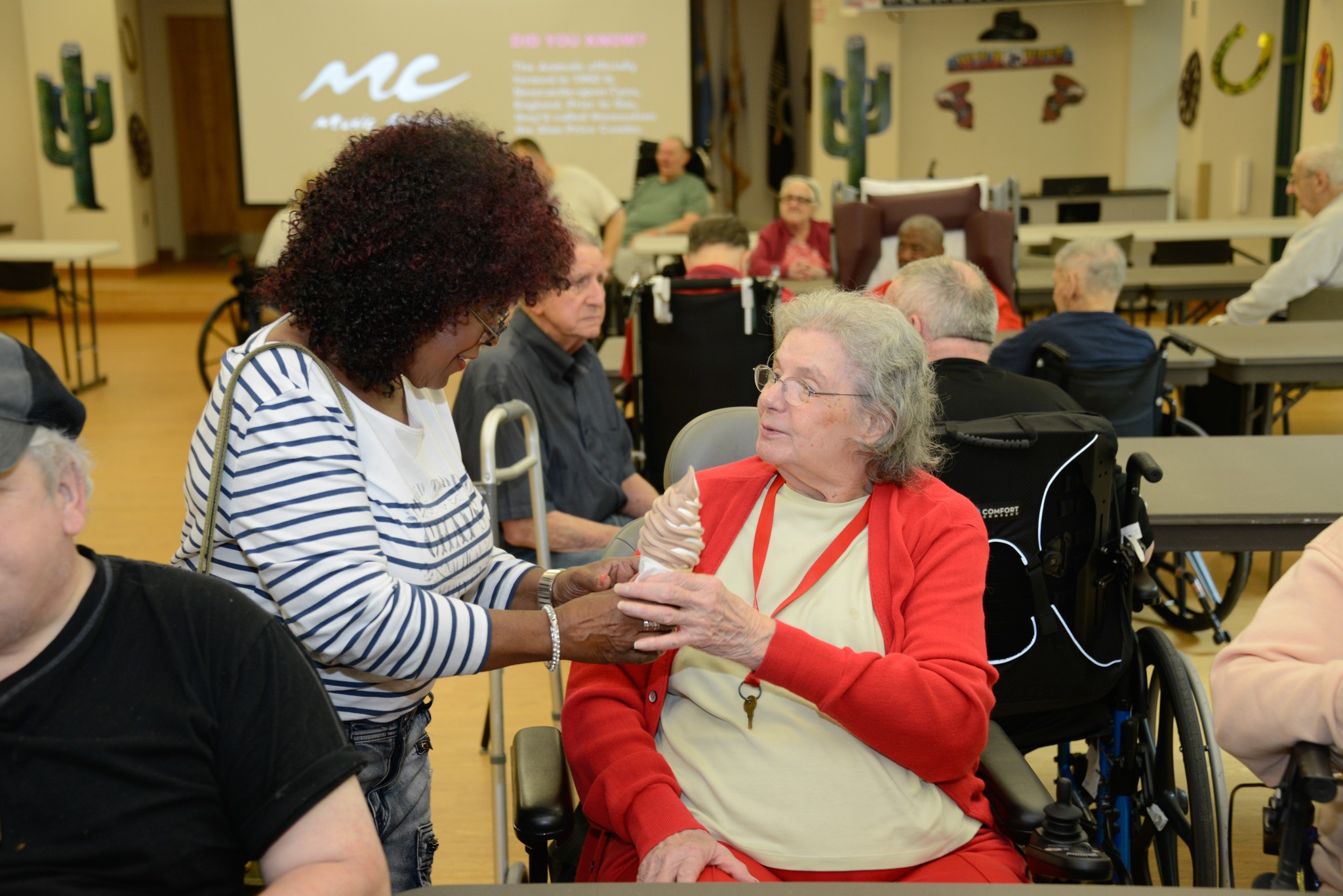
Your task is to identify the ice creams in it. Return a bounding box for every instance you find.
[637,465,705,574]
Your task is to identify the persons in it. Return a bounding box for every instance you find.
[170,115,676,892]
[561,284,1032,883]
[259,135,1167,568]
[1210,515,1343,895]
[1,332,392,896]
[1206,140,1342,324]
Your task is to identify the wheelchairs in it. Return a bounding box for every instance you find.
[509,271,1343,894]
[196,254,283,395]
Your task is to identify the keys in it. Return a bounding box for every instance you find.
[743,695,758,730]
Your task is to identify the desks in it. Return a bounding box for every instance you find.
[0,238,119,393]
[595,219,1343,553]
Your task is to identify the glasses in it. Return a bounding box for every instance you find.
[1288,172,1311,185]
[780,195,808,203]
[467,307,511,345]
[752,362,870,407]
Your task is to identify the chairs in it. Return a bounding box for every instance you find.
[1149,239,1235,325]
[1046,234,1133,318]
[0,261,70,379]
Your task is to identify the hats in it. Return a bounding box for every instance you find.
[782,174,822,207]
[0,332,85,472]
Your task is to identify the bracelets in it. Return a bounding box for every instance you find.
[537,568,565,606]
[540,604,560,672]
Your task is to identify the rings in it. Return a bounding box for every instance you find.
[643,620,660,632]
